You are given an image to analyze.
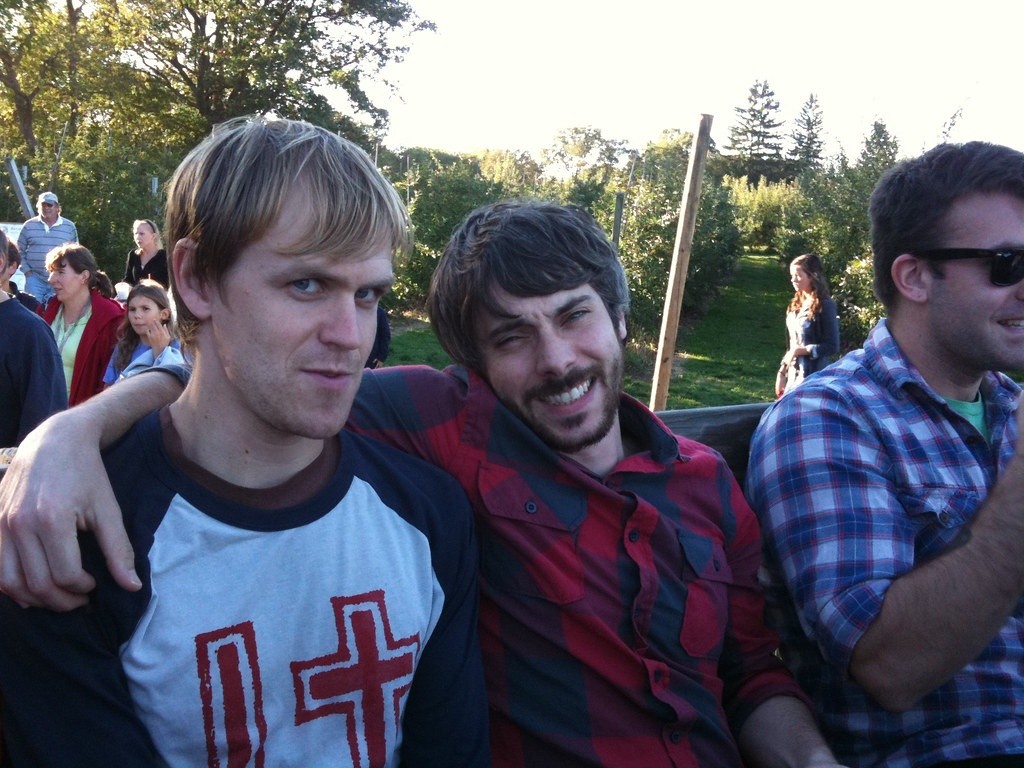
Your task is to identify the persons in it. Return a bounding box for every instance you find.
[0,112,492,768]
[0,202,847,768]
[743,141,1024,768]
[0,192,173,447]
[775,254,840,400]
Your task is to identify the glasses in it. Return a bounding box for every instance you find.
[41,203,57,207]
[915,248,1024,286]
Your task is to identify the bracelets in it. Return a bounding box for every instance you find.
[779,370,785,376]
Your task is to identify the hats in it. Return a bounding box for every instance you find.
[36,191,58,207]
